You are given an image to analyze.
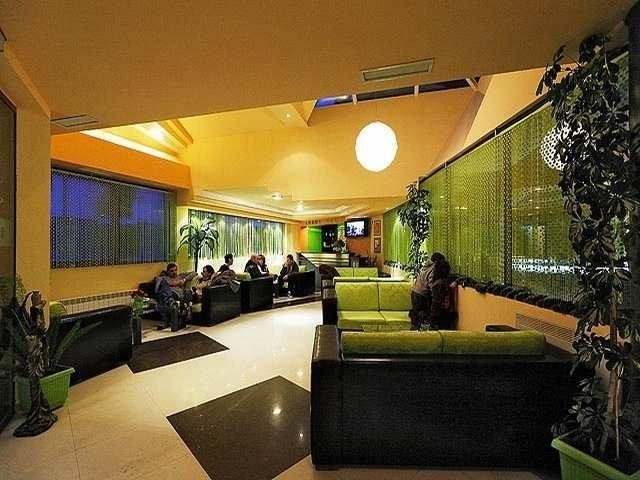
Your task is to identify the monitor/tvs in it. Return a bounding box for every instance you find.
[344,218,369,236]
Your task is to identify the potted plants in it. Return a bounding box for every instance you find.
[13,318,102,413]
[536,47,640,480]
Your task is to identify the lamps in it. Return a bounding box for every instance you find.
[541,120,593,170]
[355,121,398,173]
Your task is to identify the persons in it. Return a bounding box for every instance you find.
[219,254,233,274]
[257,256,268,275]
[273,254,299,299]
[155,263,193,332]
[191,265,216,303]
[409,252,445,330]
[427,261,459,330]
[319,264,341,280]
[244,255,270,279]
[351,226,357,235]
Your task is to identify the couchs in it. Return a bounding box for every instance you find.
[139,278,241,327]
[335,267,391,277]
[311,325,595,469]
[323,281,414,330]
[283,265,316,297]
[322,277,410,289]
[236,272,274,312]
[49,300,134,387]
